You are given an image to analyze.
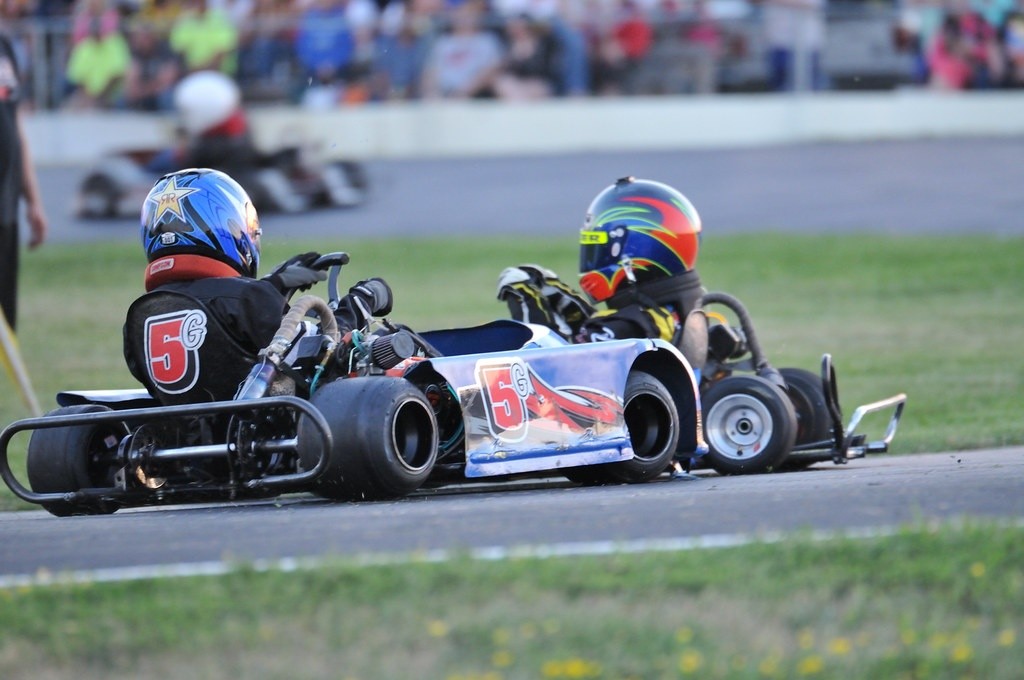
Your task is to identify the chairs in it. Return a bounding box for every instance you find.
[126,289,259,411]
[678,308,710,369]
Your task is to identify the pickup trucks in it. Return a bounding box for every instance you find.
[643,1,922,89]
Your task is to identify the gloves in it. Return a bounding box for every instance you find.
[261,252,328,297]
[497,263,559,303]
[350,278,393,317]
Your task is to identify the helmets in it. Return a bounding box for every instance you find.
[140,167,262,278]
[172,70,239,136]
[578,176,703,305]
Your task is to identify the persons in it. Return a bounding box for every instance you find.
[497,176,703,345]
[0,38,49,334]
[149,71,272,166]
[0,0,1024,117]
[139,166,445,360]
